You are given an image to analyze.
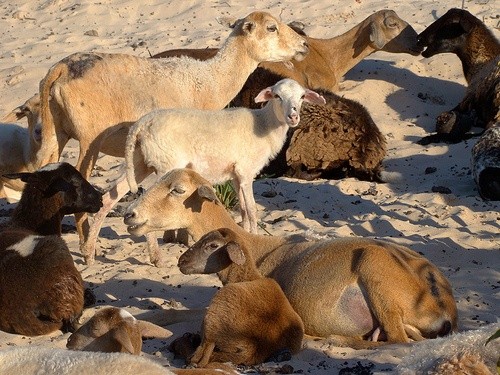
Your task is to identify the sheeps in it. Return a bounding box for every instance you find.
[0,160,103,337]
[178,227,304,368]
[34,11,310,257]
[223,67,387,185]
[1,88,43,192]
[123,161,458,350]
[149,10,424,109]
[418,8,500,130]
[0,306,241,375]
[79,77,327,268]
